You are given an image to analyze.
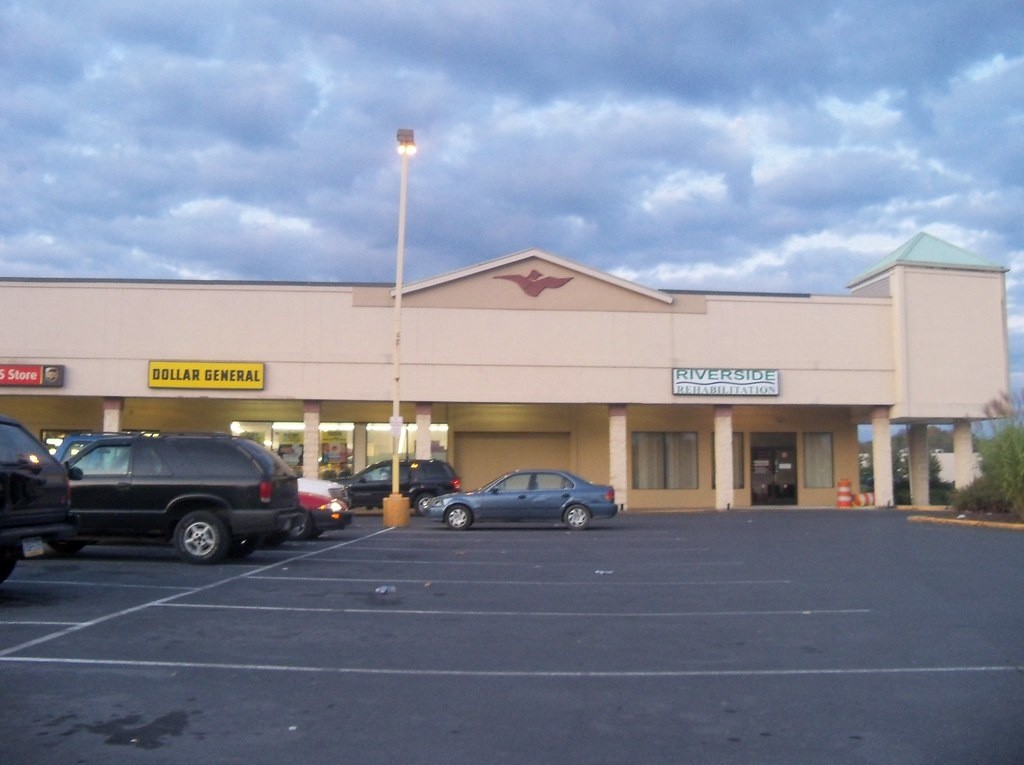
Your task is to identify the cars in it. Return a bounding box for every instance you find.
[426,469,618,530]
[54,432,136,465]
[286,490,352,539]
[297,475,352,513]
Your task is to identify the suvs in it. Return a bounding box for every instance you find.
[0,416,71,584]
[41,432,303,564]
[329,459,461,518]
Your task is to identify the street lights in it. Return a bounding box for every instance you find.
[382,122,419,526]
